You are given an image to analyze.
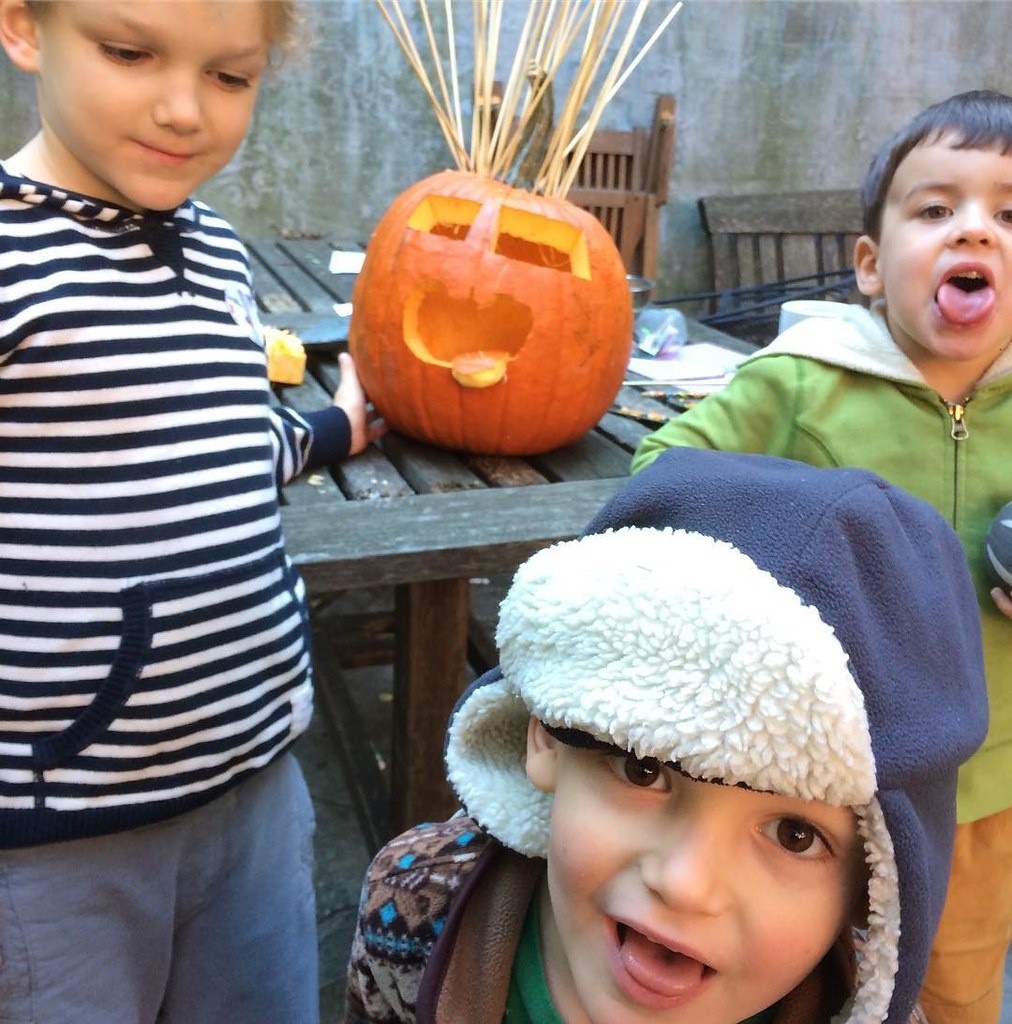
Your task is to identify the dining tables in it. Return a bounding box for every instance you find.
[237,231,779,860]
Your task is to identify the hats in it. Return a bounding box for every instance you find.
[439,445,987,1024]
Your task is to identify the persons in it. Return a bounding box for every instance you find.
[624,86,1009,1024]
[1,1,390,1024]
[343,447,990,1024]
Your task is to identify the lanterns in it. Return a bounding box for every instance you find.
[345,165,636,457]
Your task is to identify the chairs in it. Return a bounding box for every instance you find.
[703,190,876,350]
